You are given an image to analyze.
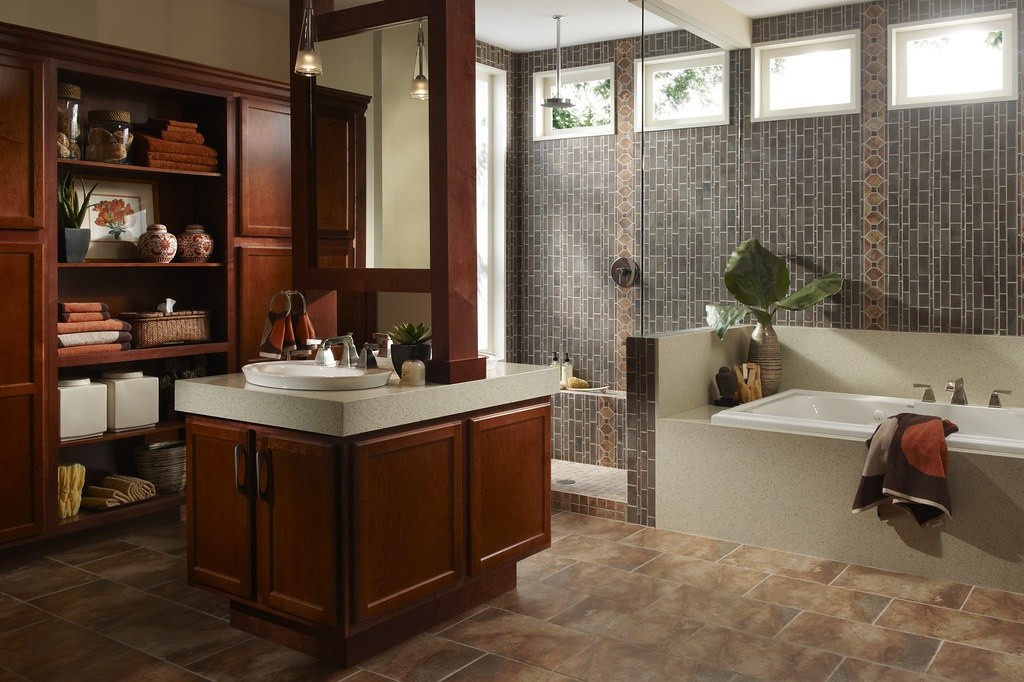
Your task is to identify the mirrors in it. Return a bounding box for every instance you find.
[309,17,430,269]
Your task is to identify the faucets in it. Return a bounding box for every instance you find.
[943,376,970,406]
[321,334,361,369]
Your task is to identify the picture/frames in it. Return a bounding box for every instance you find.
[74,175,158,263]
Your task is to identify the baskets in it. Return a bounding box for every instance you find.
[118,310,210,348]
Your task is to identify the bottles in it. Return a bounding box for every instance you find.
[56,81,81,161]
[88,109,130,165]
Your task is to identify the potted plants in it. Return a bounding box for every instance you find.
[706,238,844,399]
[388,322,432,380]
[57,169,98,263]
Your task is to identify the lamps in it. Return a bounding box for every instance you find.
[293,0,323,77]
[410,21,428,100]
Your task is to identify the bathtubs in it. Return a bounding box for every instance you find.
[728,385,1024,463]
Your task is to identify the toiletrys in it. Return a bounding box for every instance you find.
[550,351,572,386]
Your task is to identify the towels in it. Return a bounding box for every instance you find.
[258,309,319,359]
[128,117,219,173]
[55,301,133,356]
[850,411,960,528]
[77,474,157,508]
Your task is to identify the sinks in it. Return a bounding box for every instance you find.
[241,359,376,384]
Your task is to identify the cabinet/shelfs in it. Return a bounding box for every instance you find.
[0,20,552,669]
